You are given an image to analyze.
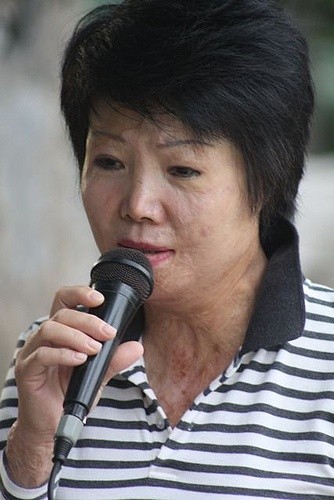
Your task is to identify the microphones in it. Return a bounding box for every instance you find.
[48,250,156,462]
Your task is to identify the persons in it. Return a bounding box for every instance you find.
[0,1,334,500]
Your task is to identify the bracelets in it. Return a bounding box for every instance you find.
[1,445,61,500]
